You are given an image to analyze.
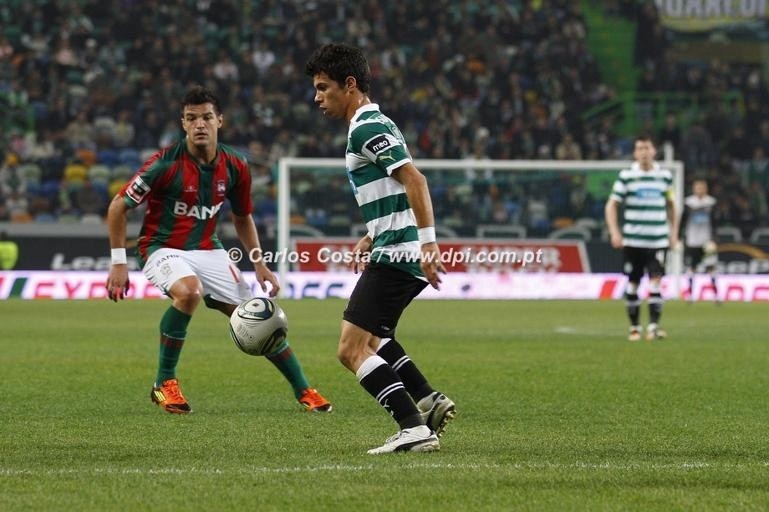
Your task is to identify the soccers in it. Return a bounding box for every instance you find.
[228,297,288,356]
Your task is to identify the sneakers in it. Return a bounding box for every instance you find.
[419,392,457,441]
[365,428,441,456]
[150,378,194,414]
[298,386,334,414]
[627,326,667,342]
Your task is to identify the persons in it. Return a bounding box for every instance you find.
[105,84,336,415]
[305,41,457,456]
[678,177,722,304]
[606,137,682,341]
